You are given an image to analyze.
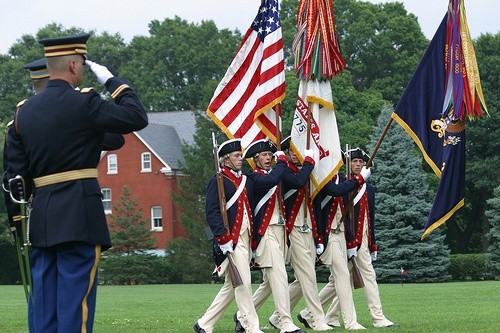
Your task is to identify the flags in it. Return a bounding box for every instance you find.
[206,0,287,172]
[289,80,345,205]
[391,10,464,240]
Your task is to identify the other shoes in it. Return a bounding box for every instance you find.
[279,323,306,333]
[347,323,367,330]
[233,311,245,333]
[268,310,279,329]
[297,309,313,328]
[192,322,206,333]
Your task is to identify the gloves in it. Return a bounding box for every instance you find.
[348,246,358,259]
[220,240,234,255]
[272,150,285,163]
[316,243,324,255]
[370,251,377,261]
[361,166,372,182]
[84,60,114,85]
[304,148,315,158]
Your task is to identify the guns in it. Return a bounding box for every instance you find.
[346,142,366,289]
[211,131,244,289]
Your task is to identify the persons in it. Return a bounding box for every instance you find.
[3,35,149,333]
[192,137,400,333]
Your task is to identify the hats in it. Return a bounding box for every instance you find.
[23,57,49,79]
[39,33,91,60]
[344,147,374,167]
[279,135,291,150]
[243,138,278,158]
[217,138,242,157]
